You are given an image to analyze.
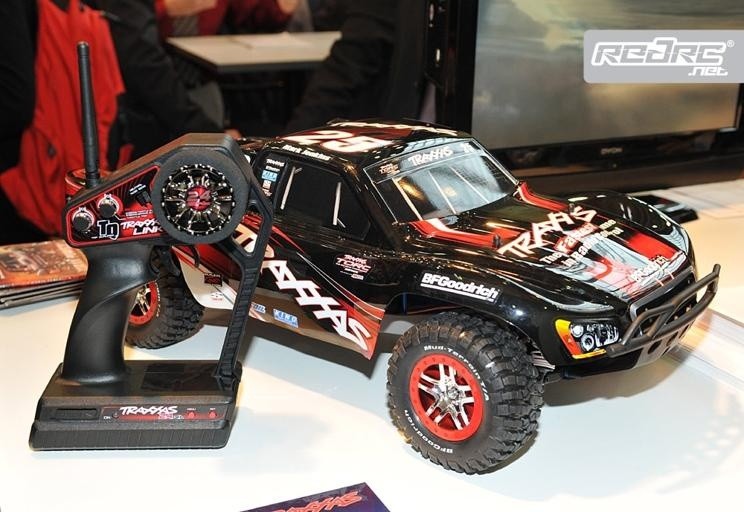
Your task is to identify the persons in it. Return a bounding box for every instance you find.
[282,1,437,138]
[1,1,240,249]
[153,0,307,137]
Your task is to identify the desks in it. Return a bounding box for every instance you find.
[0,176,743,503]
[164,27,343,130]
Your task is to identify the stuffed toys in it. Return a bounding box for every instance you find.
[25,37,722,476]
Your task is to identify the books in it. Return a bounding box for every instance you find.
[0,240,88,312]
